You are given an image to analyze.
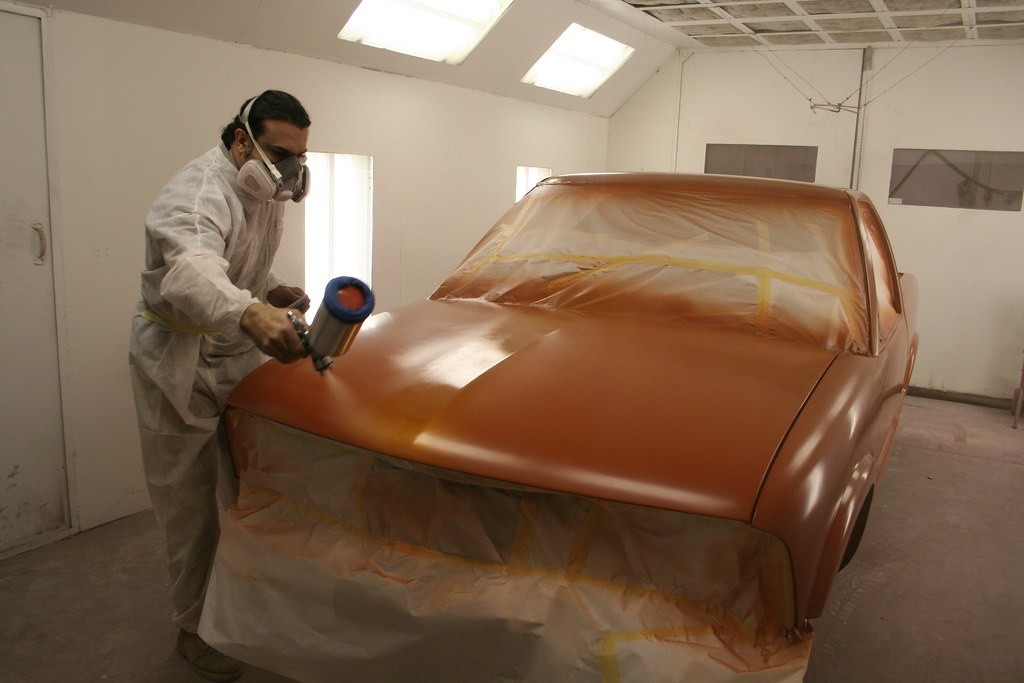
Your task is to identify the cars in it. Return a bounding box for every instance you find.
[213,172,920,681]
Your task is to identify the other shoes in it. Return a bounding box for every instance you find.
[176,628,246,683]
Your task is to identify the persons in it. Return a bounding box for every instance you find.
[129,90,312,683]
